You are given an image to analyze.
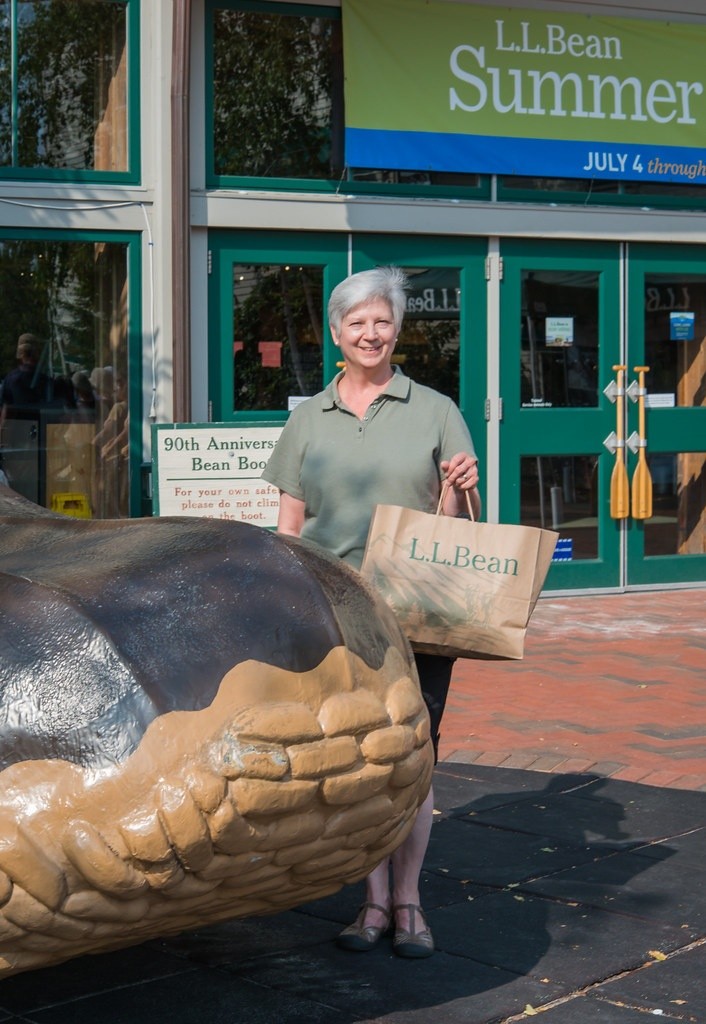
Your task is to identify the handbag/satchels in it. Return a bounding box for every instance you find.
[358,476,560,664]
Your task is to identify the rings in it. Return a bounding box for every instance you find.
[461,472,469,481]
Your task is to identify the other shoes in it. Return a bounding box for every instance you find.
[392,904,434,960]
[340,902,393,952]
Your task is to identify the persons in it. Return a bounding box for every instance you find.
[0,331,132,519]
[261,265,482,963]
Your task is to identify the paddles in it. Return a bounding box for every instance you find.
[632,365,652,520]
[608,365,630,520]
